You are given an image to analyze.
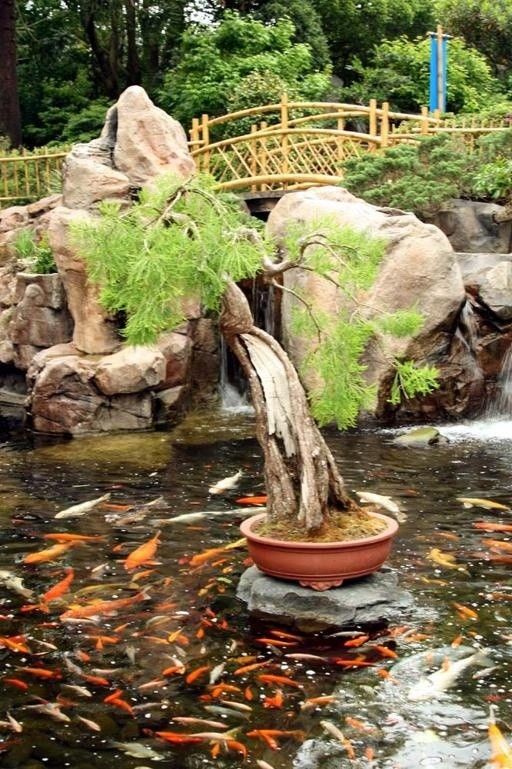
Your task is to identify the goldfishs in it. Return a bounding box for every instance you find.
[0,467,512,768]
